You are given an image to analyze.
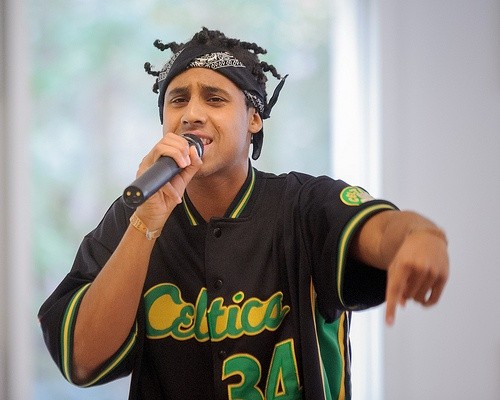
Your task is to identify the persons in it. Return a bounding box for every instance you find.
[37,26,449,400]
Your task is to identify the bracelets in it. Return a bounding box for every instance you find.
[129,213,161,240]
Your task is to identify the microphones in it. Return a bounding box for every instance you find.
[124,134,204,209]
